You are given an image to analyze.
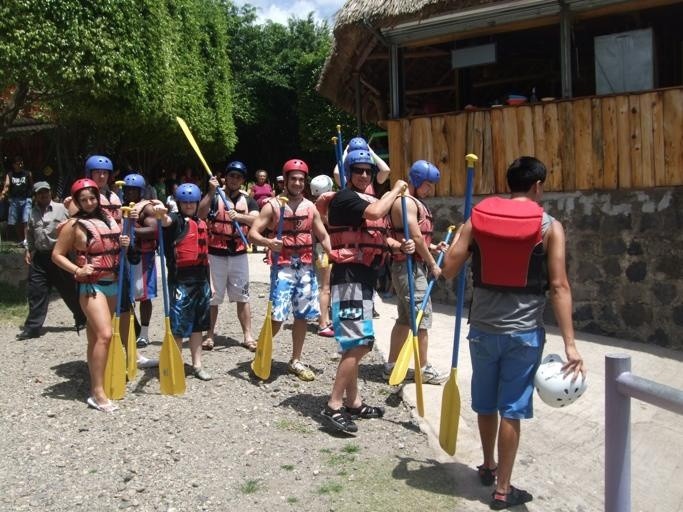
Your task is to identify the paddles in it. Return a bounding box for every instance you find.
[398,184,425,419]
[126,202,138,384]
[439,151,479,456]
[176,117,253,254]
[156,210,187,396]
[103,206,132,401]
[254,196,289,380]
[388,224,455,388]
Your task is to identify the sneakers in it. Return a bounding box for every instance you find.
[317,327,335,337]
[327,323,333,329]
[372,310,379,319]
[137,356,160,368]
[419,363,450,385]
[343,405,385,419]
[383,363,414,381]
[191,366,213,381]
[490,485,532,510]
[137,337,149,347]
[317,402,358,432]
[288,360,315,381]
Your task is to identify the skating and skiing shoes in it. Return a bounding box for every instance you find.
[476,465,499,485]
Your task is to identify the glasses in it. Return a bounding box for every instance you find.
[349,167,372,176]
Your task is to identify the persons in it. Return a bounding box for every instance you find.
[142,174,157,200]
[310,174,335,337]
[151,182,216,381]
[248,160,331,381]
[153,174,169,207]
[166,183,179,214]
[249,169,274,253]
[320,150,415,437]
[382,160,451,385]
[168,172,177,193]
[121,173,159,348]
[273,176,285,196]
[195,453,202,460]
[441,156,586,510]
[182,167,195,184]
[63,155,138,369]
[333,137,390,197]
[16,181,86,340]
[51,178,130,414]
[196,162,259,353]
[0,155,33,248]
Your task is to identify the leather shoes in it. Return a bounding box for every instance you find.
[72,324,86,330]
[16,330,40,339]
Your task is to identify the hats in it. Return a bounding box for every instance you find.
[33,181,51,192]
[276,176,284,180]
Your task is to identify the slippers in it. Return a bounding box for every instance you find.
[241,341,257,352]
[87,397,120,412]
[201,340,215,350]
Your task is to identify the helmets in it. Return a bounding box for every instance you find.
[176,183,201,202]
[226,161,246,174]
[123,174,145,197]
[343,150,376,176]
[311,175,334,196]
[409,160,441,188]
[70,179,100,210]
[86,155,113,179]
[533,354,588,407]
[346,138,369,153]
[283,160,308,177]
